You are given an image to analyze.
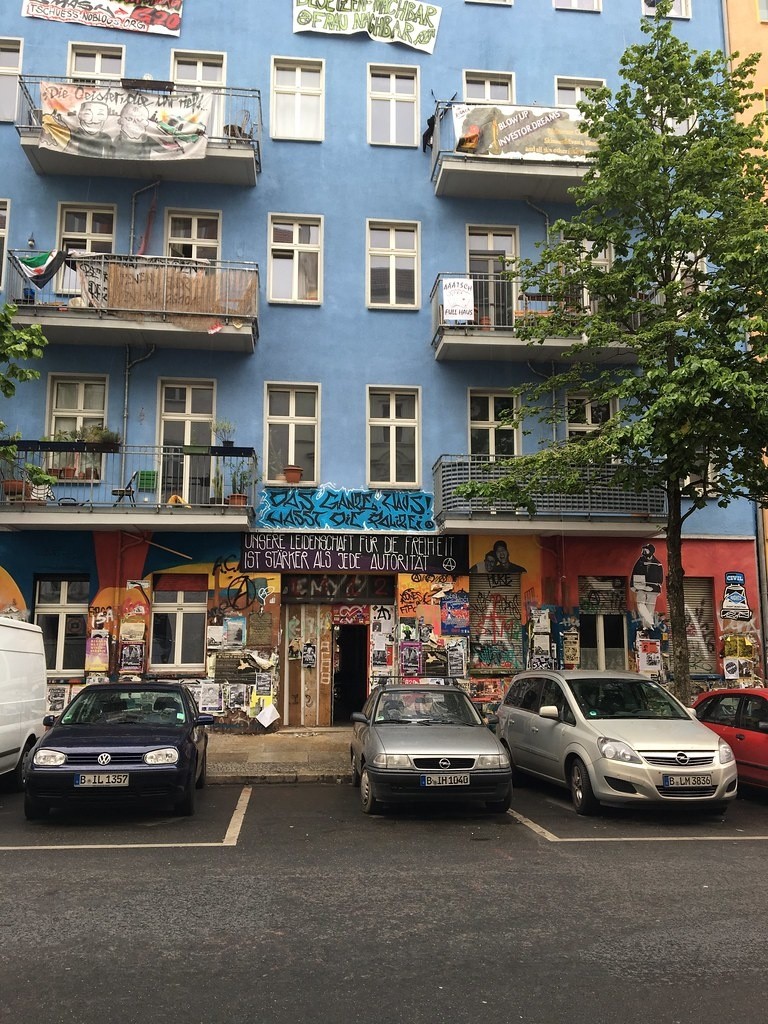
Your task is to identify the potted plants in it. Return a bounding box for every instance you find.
[86,425,112,479]
[211,418,236,448]
[270,430,286,481]
[62,430,79,478]
[41,436,62,477]
[1,433,30,496]
[227,461,251,507]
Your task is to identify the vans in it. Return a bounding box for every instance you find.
[0,616,52,792]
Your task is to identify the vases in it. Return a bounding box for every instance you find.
[283,465,303,483]
[470,307,491,331]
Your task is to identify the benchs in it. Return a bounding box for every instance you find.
[515,292,581,330]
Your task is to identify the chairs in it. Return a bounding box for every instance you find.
[719,708,768,730]
[92,697,183,725]
[59,498,89,506]
[531,686,642,718]
[112,472,138,506]
[378,698,461,721]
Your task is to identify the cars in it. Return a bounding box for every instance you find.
[348,683,514,819]
[23,681,215,824]
[689,686,768,799]
[496,666,737,817]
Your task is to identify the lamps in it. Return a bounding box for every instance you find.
[26,232,35,246]
